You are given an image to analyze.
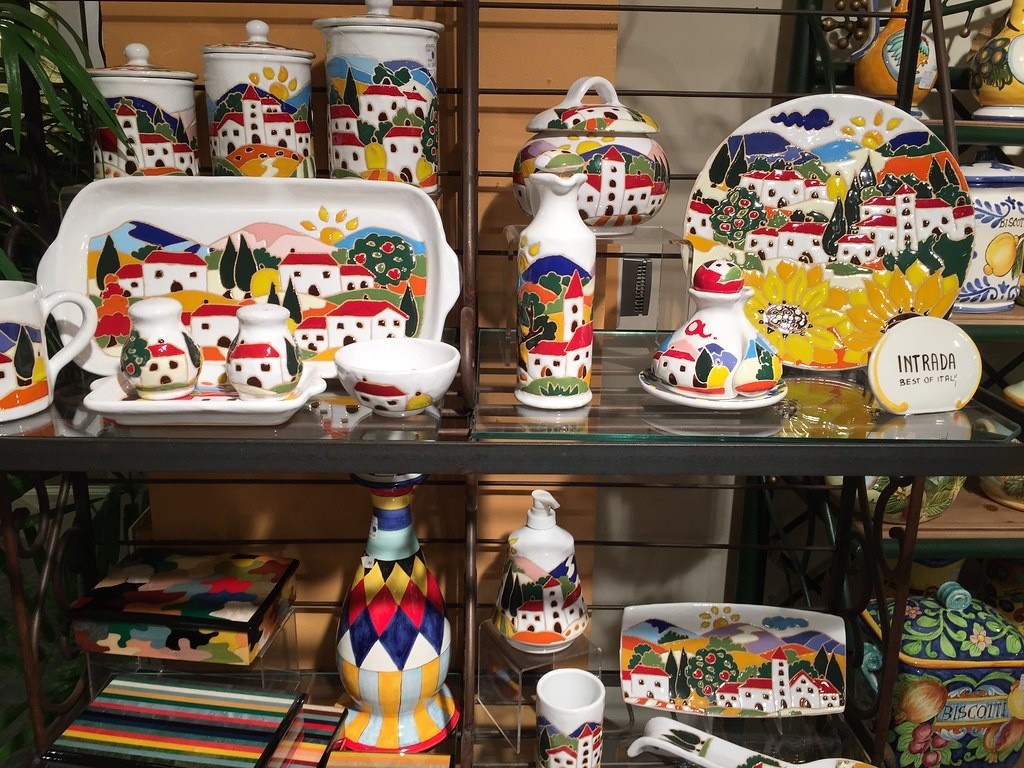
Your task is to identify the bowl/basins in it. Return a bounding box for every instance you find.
[334,338,460,416]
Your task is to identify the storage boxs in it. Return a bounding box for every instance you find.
[70,546,298,664]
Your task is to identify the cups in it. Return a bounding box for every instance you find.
[200,19,316,178]
[225,304,304,401]
[536,668,606,768]
[82,42,197,178]
[121,297,203,400]
[949,162,1024,314]
[0,280,99,423]
[312,0,446,193]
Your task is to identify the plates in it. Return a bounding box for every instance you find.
[867,316,982,415]
[627,716,879,768]
[681,93,975,371]
[620,602,846,719]
[639,370,789,409]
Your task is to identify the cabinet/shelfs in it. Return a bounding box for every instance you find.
[0,0,1024,768]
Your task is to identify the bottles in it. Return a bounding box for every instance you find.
[336,472,460,753]
[650,263,783,400]
[969,0,1024,119]
[515,174,597,412]
[853,0,938,120]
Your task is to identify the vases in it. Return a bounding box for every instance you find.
[335,472,461,755]
